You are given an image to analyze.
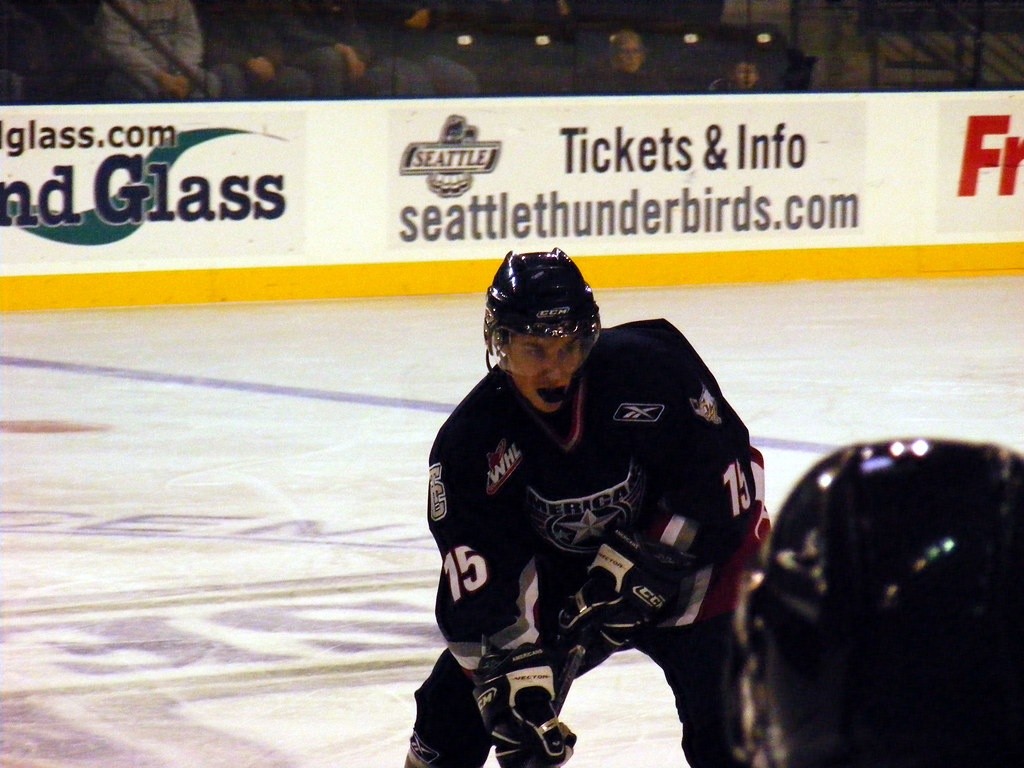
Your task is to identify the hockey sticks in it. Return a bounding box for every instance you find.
[515,644,585,768]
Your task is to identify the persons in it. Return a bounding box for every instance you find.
[404,245,775,768]
[730,436,1024,767]
[99,0,897,91]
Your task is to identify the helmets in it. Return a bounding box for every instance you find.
[744,438,1024,768]
[482,247,599,355]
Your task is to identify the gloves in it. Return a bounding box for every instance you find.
[470,642,576,768]
